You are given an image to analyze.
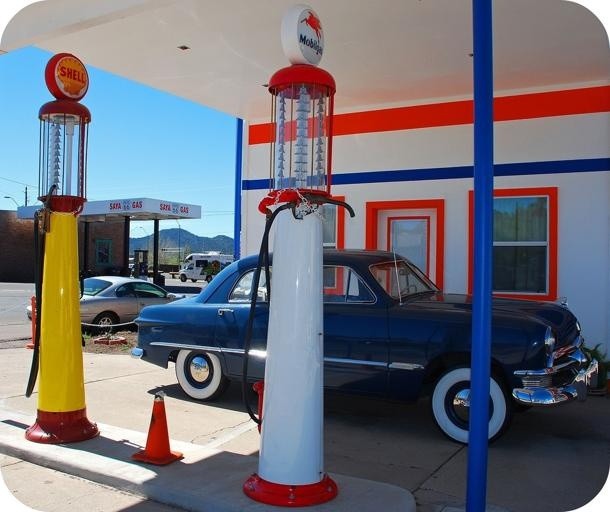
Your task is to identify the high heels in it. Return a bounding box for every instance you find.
[130,392,183,466]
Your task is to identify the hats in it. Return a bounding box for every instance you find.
[25,274,185,335]
[134,248,598,443]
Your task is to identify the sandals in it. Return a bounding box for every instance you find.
[180,252,233,282]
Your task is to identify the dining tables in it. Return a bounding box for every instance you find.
[584,342,609,390]
